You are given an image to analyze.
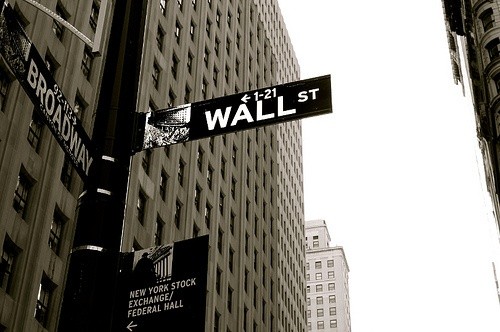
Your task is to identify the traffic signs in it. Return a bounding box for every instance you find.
[0,0,335,332]
